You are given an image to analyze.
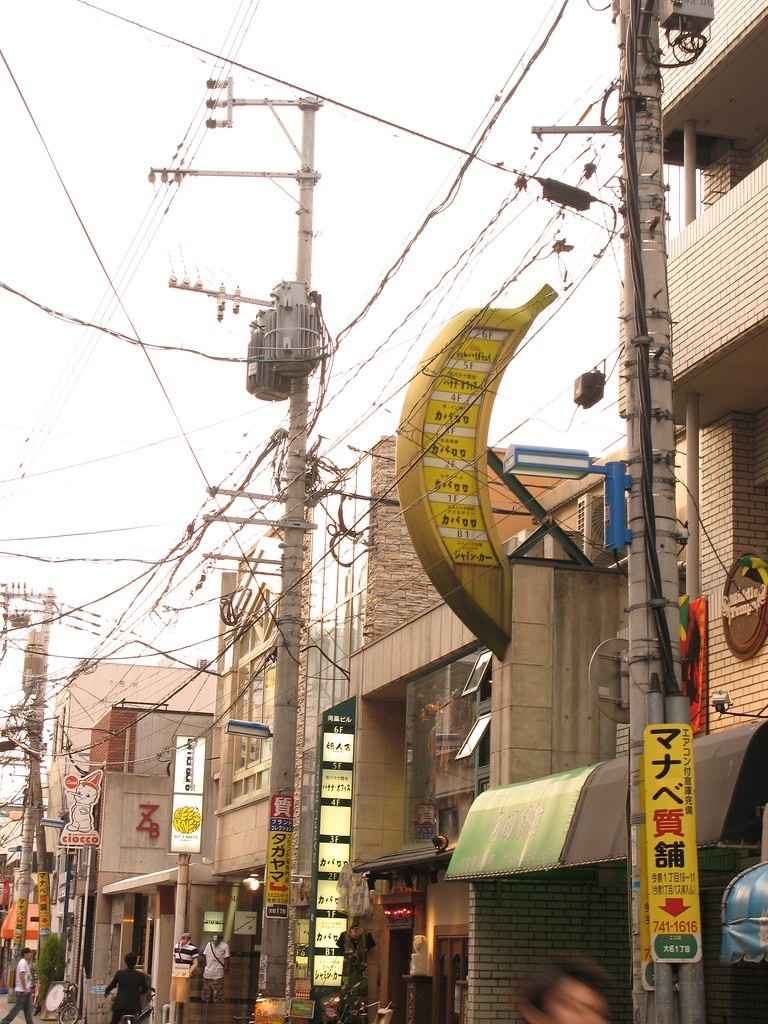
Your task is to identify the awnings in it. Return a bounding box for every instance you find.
[444,719,768,965]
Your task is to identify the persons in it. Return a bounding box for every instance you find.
[104,952,148,1024]
[0,948,34,1024]
[516,963,609,1024]
[169,933,229,1003]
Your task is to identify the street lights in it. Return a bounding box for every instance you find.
[39,817,73,984]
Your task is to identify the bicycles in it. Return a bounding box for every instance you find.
[104,990,149,1024]
[56,980,80,1024]
